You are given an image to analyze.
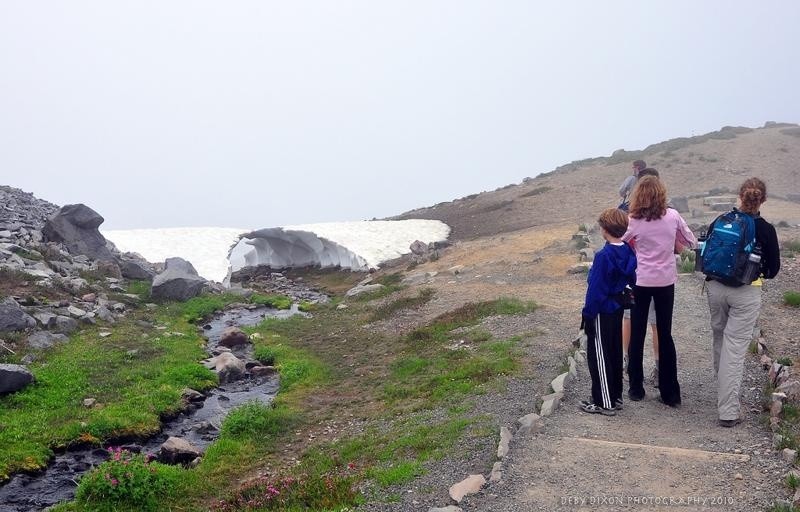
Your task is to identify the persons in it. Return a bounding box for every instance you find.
[578,206,638,416]
[619,159,647,200]
[697,176,782,428]
[616,167,663,388]
[620,174,699,408]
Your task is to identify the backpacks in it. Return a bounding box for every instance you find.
[700,207,767,289]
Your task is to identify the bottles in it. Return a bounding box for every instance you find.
[740,243,763,285]
[695,230,706,272]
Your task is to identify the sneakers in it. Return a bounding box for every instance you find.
[719,414,745,427]
[579,396,624,416]
[623,369,681,408]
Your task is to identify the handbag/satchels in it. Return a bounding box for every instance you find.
[618,202,629,211]
